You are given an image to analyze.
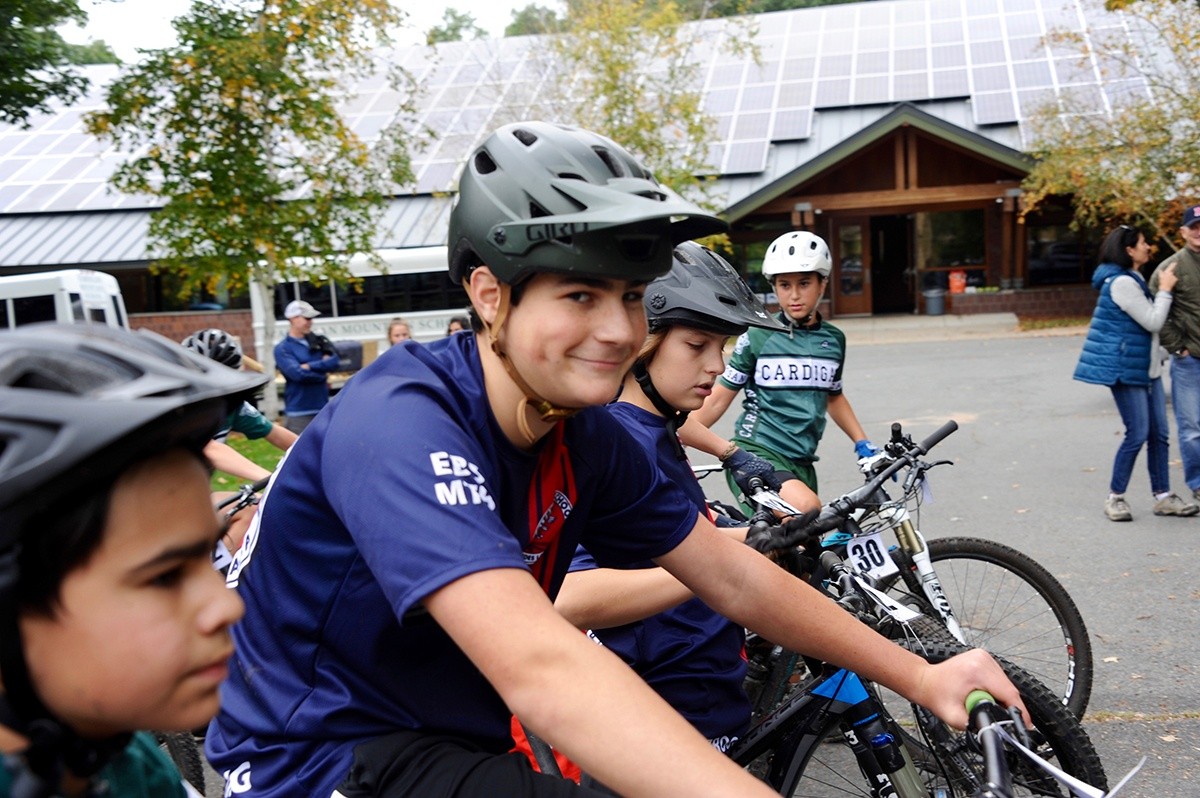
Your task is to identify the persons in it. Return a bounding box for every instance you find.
[691,231,877,530]
[521,242,822,798]
[195,121,1033,798]
[1069,223,1200,522]
[0,321,271,798]
[181,299,471,590]
[1148,204,1200,502]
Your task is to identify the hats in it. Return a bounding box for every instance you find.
[284,300,321,318]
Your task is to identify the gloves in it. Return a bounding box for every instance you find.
[722,448,782,498]
[854,440,879,460]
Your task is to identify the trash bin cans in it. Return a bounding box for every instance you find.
[922,289,945,315]
[948,270,967,293]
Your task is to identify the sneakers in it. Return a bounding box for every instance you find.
[1103,494,1132,521]
[1193,488,1200,499]
[1154,493,1200,517]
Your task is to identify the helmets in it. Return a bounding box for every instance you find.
[181,328,243,370]
[0,321,272,501]
[642,240,794,335]
[762,231,832,288]
[1182,206,1200,228]
[449,120,730,286]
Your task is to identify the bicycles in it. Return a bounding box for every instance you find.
[212,473,273,520]
[686,419,1150,798]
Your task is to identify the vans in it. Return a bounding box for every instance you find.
[0,269,132,333]
